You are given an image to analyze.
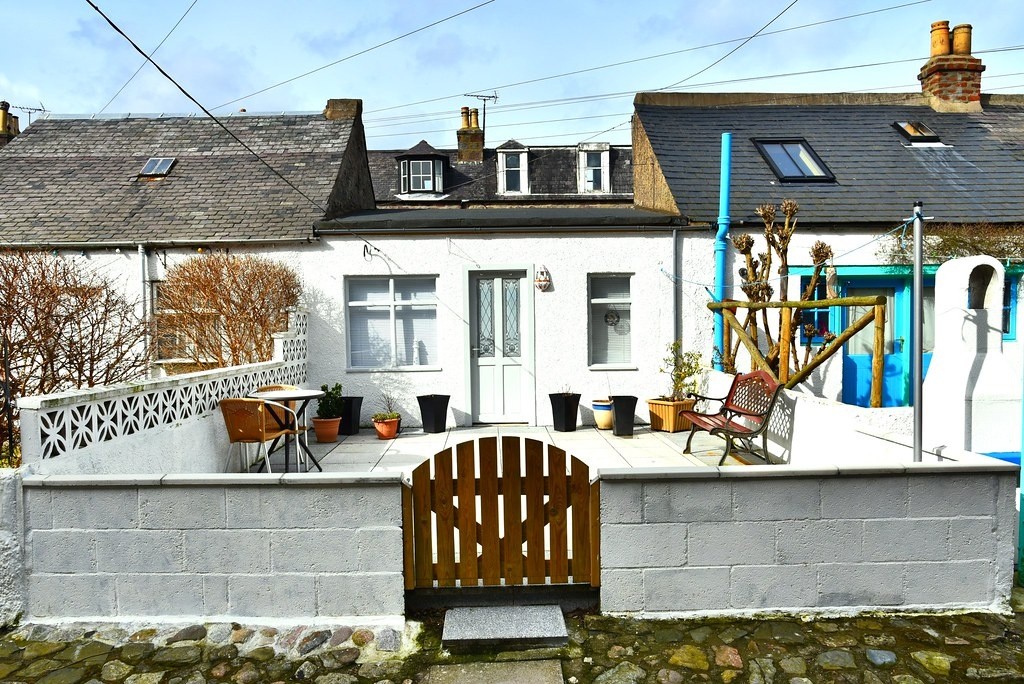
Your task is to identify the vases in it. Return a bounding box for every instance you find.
[338,395,364,436]
[608,395,638,436]
[592,400,613,429]
[417,394,450,433]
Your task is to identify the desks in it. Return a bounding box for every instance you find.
[247,389,326,473]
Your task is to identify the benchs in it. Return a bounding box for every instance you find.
[679,368,785,466]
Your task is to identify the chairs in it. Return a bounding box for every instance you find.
[250,384,309,472]
[219,398,300,473]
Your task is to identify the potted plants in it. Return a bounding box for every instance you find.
[645,338,704,433]
[549,385,582,432]
[372,411,400,440]
[377,390,403,433]
[310,383,343,443]
